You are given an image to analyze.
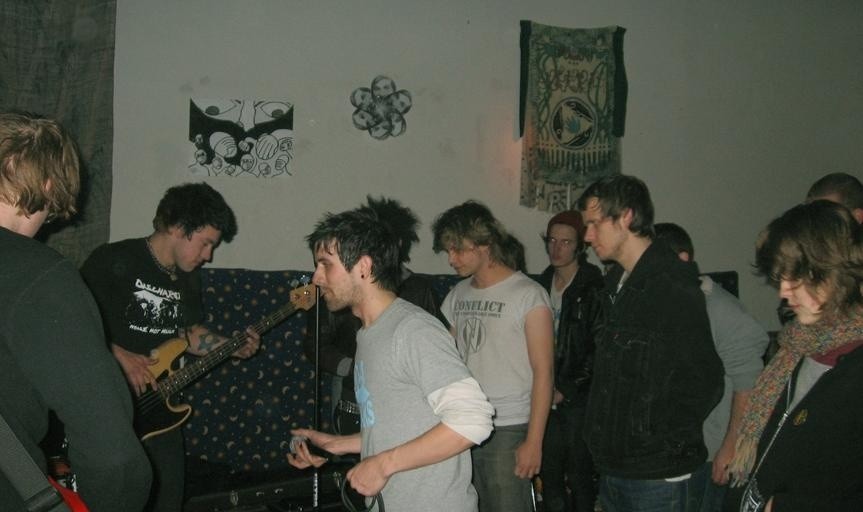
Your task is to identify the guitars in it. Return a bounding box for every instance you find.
[52,274,320,477]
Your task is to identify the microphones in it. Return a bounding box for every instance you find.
[289,434,353,472]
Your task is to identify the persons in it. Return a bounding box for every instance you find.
[489,228,531,275]
[281,206,496,512]
[0,106,155,512]
[803,171,861,227]
[428,198,557,512]
[532,209,602,512]
[39,181,263,512]
[314,194,460,512]
[648,220,770,510]
[721,195,863,512]
[548,170,727,510]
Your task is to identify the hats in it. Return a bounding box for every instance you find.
[548,210,589,253]
